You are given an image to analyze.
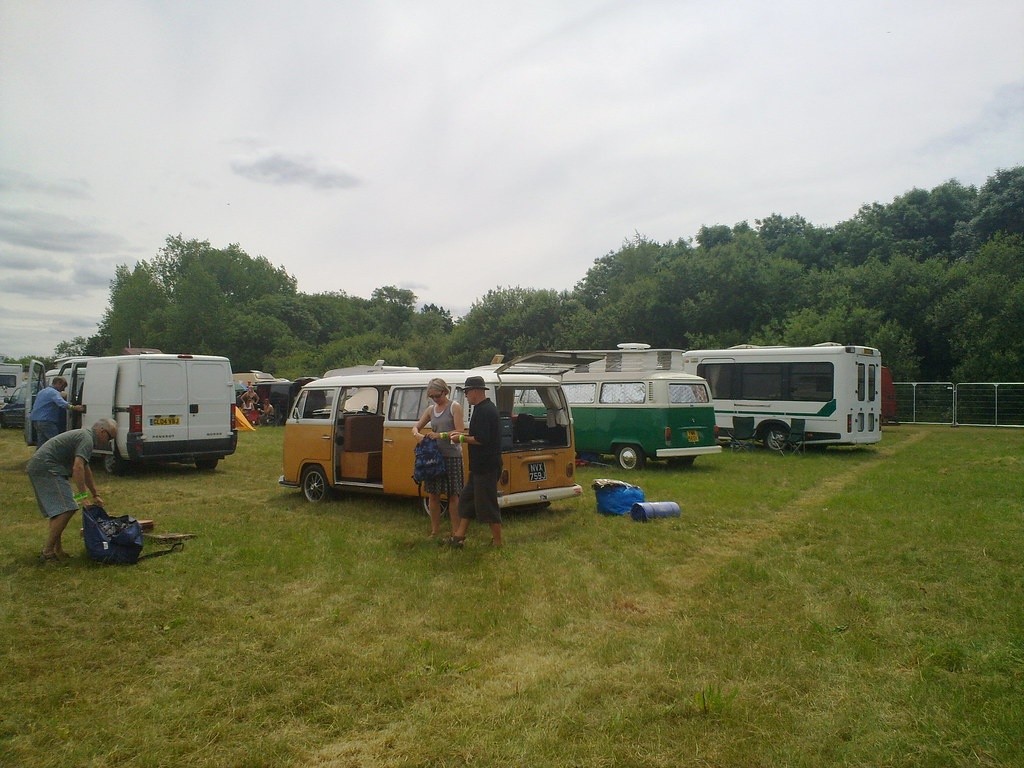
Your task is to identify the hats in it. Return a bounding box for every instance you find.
[456,376,490,390]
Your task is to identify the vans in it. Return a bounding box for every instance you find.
[684,342,882,454]
[511,368,722,474]
[23,351,238,478]
[0,356,98,429]
[233,360,430,424]
[279,350,609,521]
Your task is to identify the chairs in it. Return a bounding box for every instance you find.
[727,416,757,452]
[773,418,805,456]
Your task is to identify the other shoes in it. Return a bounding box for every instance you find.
[429,529,437,539]
[439,532,465,549]
[38,549,82,569]
[481,538,503,549]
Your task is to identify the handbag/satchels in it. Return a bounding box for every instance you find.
[596,486,645,516]
[81,504,184,564]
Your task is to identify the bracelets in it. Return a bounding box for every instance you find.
[259,409,261,412]
[459,435,464,443]
[440,432,448,440]
[93,494,100,498]
[413,432,419,437]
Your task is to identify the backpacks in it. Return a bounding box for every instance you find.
[411,434,446,485]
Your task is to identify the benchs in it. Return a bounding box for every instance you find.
[342,415,384,479]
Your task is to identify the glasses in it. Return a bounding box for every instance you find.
[102,427,114,441]
[429,390,444,398]
[465,389,471,395]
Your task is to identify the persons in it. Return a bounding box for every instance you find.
[27,418,118,569]
[412,378,464,544]
[258,399,275,426]
[436,376,504,550]
[30,377,84,452]
[240,386,260,426]
